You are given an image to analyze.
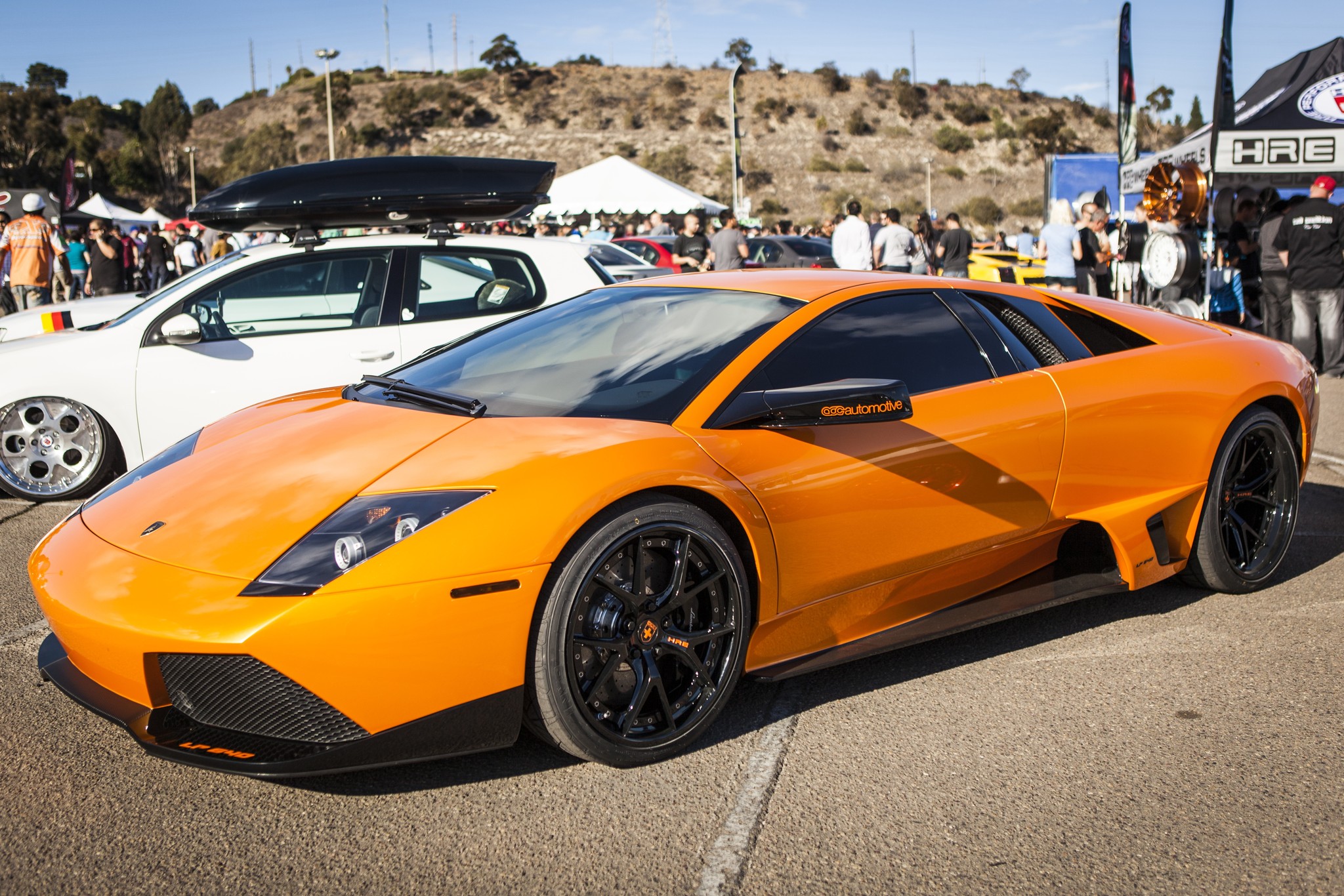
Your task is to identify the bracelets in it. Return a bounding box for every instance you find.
[96,238,102,243]
[85,281,90,284]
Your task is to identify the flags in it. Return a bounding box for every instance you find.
[60,148,80,234]
[1118,2,1140,164]
[1211,0,1234,174]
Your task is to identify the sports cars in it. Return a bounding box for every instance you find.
[28,266,1321,778]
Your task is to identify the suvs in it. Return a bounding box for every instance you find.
[0,155,672,504]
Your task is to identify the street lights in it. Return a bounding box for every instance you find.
[183,146,196,210]
[315,48,341,162]
[921,157,943,216]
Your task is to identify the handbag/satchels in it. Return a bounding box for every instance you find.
[927,265,936,277]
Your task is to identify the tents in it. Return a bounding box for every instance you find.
[1120,35,1344,321]
[76,193,158,235]
[164,217,205,230]
[533,154,728,217]
[143,206,172,230]
[0,188,113,230]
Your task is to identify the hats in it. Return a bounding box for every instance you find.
[22,193,46,212]
[590,219,600,231]
[1314,176,1336,194]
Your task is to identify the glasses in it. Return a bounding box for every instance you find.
[89,228,102,233]
[1099,219,1108,227]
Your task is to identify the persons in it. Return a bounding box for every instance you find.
[319,227,394,238]
[0,193,91,316]
[84,217,206,297]
[993,224,1033,255]
[832,198,974,278]
[1038,176,1344,378]
[585,212,845,256]
[201,228,279,263]
[453,220,583,238]
[672,208,748,273]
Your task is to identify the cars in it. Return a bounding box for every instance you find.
[545,236,674,283]
[0,254,496,345]
[610,235,763,275]
[935,243,1048,289]
[742,236,840,269]
